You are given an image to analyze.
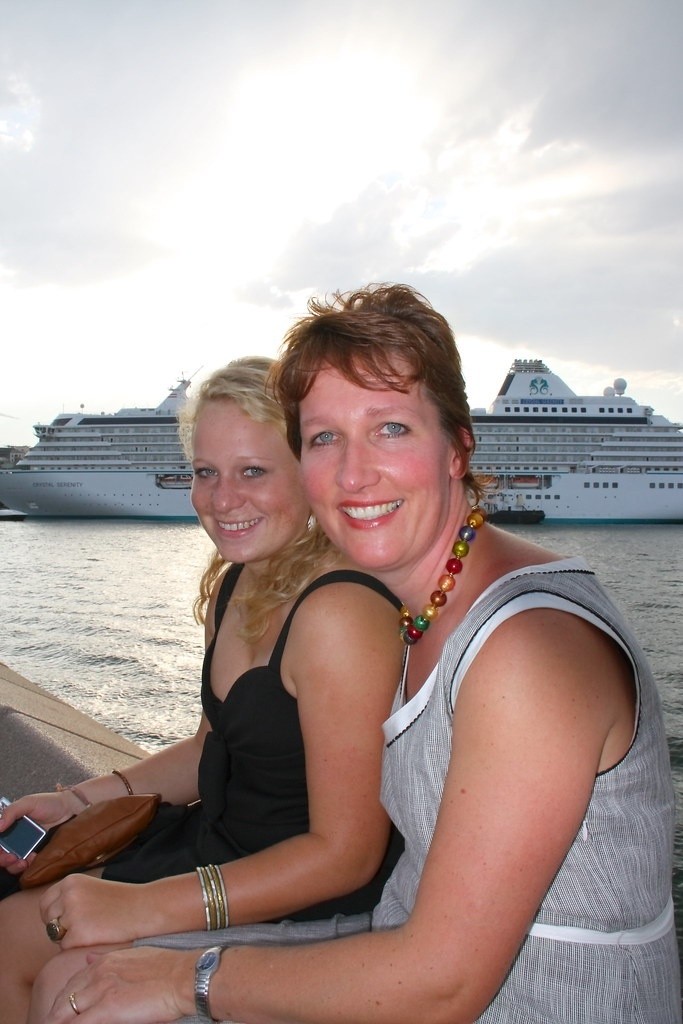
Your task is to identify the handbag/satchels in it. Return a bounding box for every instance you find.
[19,794,159,889]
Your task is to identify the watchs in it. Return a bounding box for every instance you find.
[193,938,243,1024]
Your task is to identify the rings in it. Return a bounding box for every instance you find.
[46,919,67,941]
[69,994,80,1015]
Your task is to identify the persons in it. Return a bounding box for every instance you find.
[0,284,683,1024]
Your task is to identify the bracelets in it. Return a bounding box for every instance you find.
[112,769,134,795]
[196,865,229,932]
[55,782,93,808]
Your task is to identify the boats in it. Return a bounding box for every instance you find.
[484,505,547,524]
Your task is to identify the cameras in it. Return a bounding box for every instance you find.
[0,796,48,861]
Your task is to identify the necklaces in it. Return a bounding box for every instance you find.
[398,505,486,644]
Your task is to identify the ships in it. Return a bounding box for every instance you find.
[1,358,683,525]
